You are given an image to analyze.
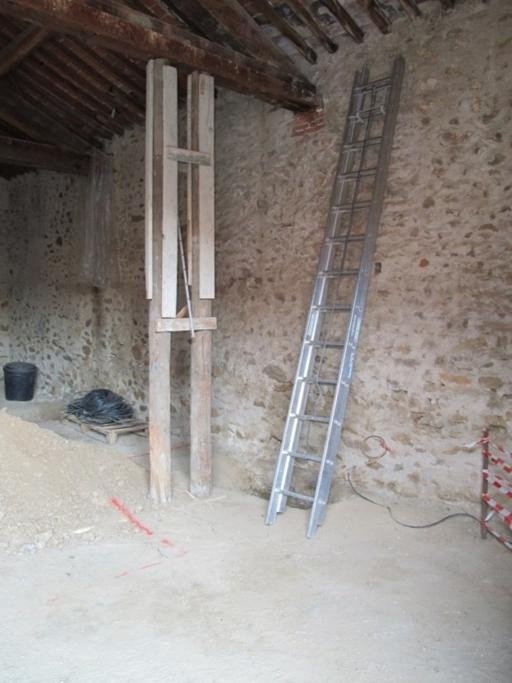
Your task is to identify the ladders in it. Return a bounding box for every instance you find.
[266,55,406,537]
